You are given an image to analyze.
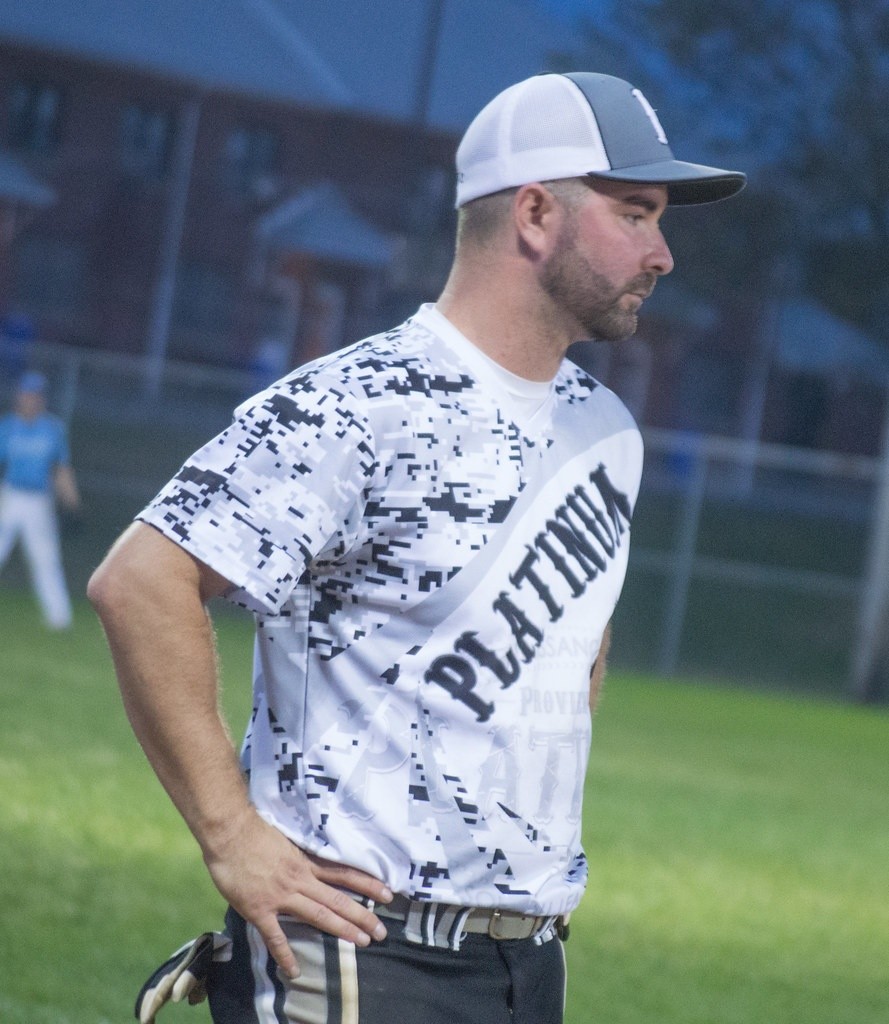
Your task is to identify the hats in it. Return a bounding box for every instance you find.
[456,71,748,208]
[12,371,50,393]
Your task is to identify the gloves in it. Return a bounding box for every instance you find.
[135,932,234,1024]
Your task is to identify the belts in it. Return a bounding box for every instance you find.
[374,890,559,942]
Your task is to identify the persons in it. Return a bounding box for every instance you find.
[85,72,745,1024]
[0,370,80,631]
